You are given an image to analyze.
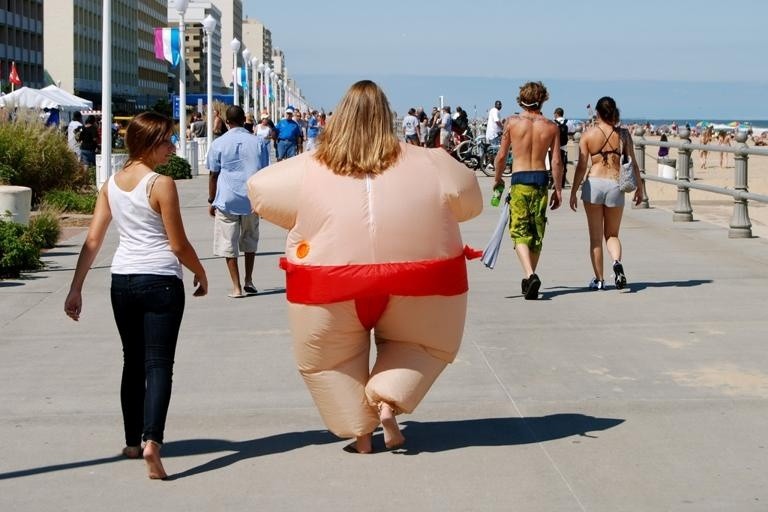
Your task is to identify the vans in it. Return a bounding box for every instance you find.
[112,116,133,147]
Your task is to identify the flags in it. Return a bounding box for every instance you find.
[9,65,21,86]
[154,26,180,69]
[231,64,249,89]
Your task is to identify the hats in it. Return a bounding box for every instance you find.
[261,114,270,120]
[286,109,293,113]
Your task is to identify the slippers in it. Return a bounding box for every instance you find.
[244,286,257,293]
[227,294,243,297]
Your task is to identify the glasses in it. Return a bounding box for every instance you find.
[519,102,539,110]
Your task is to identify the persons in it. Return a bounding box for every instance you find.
[494,80,565,301]
[204,104,271,296]
[61,109,210,481]
[568,92,647,291]
[66,99,768,186]
[243,78,485,454]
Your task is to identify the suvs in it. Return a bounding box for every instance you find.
[61,109,113,149]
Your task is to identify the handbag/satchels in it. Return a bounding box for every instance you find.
[619,154,636,193]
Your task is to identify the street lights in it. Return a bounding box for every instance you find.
[203,12,217,168]
[231,37,241,109]
[257,63,265,112]
[251,56,258,122]
[241,48,251,115]
[172,0,192,159]
[264,66,313,126]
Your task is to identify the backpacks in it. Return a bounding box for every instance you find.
[554,119,568,146]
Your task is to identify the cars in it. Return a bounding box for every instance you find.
[456,127,512,178]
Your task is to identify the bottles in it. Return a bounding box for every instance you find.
[490,181,504,206]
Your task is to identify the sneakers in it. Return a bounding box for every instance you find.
[589,278,605,292]
[525,274,541,300]
[522,278,528,293]
[613,262,626,290]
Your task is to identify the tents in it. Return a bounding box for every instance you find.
[0,82,94,129]
